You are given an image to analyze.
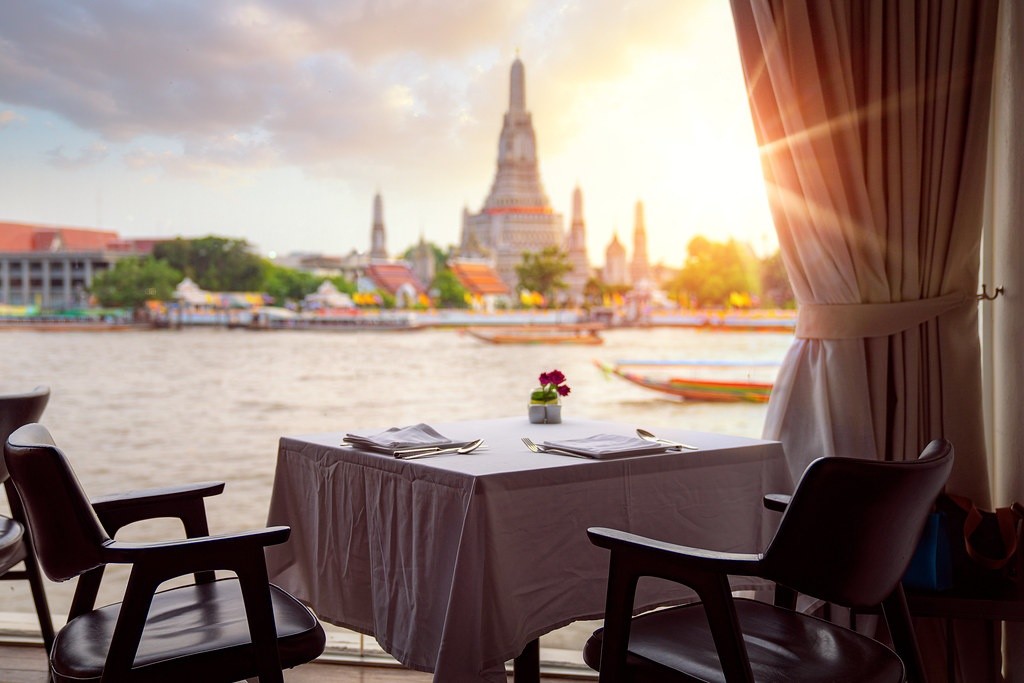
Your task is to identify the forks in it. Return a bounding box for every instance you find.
[521,437,592,459]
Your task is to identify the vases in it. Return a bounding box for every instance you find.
[531,391,558,404]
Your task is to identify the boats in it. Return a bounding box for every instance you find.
[593,357,781,403]
[460,321,604,346]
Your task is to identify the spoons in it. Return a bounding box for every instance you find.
[636,428,698,449]
[402,438,485,461]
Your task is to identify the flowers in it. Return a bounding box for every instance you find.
[540,369,571,398]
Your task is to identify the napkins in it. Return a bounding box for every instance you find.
[544,432,661,453]
[343,423,452,448]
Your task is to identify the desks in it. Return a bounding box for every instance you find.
[849,593,1024,683]
[264,417,824,683]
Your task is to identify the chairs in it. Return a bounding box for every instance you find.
[585,440,956,683]
[0,389,326,683]
